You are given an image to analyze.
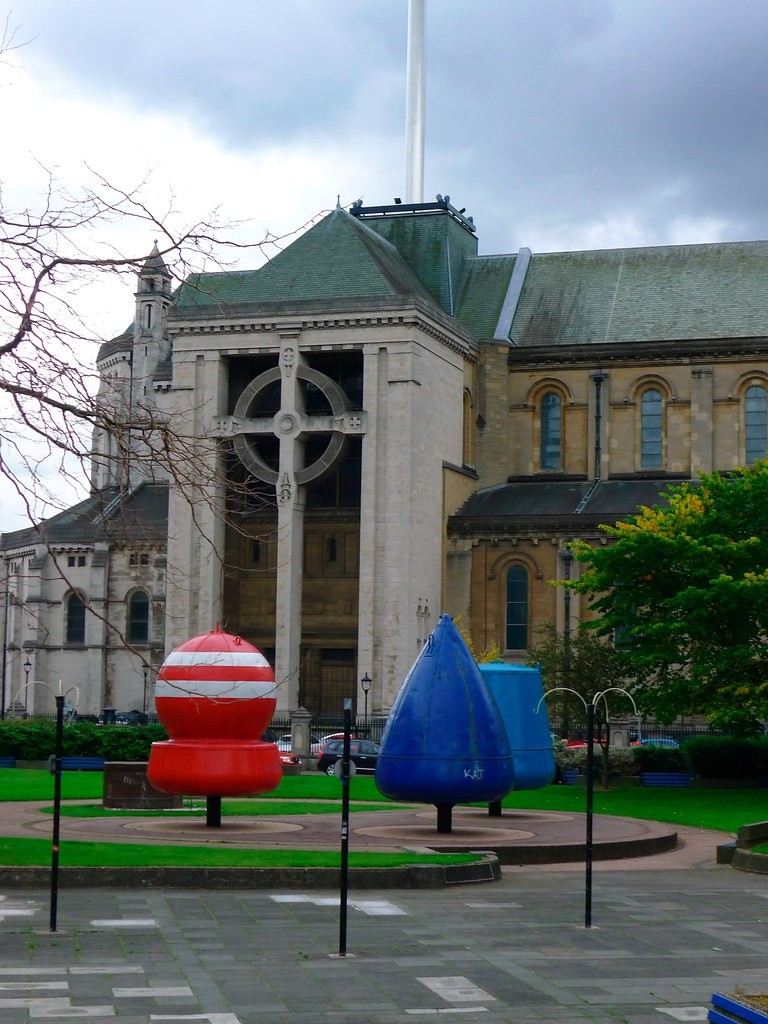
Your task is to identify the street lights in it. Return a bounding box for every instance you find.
[141,661,149,714]
[362,671,372,740]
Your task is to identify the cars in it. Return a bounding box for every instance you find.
[273,735,323,755]
[317,739,381,777]
[321,733,380,747]
[561,734,682,752]
[279,751,302,770]
